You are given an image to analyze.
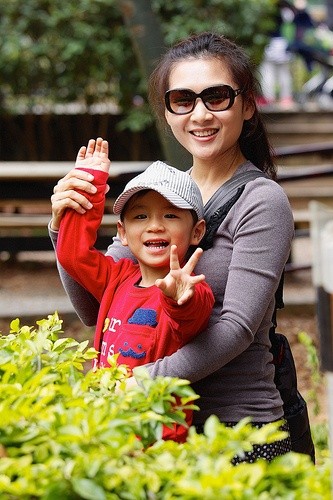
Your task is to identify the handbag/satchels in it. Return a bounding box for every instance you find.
[267,334,315,467]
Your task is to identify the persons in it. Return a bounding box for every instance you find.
[48,30,295,466]
[54,137,216,443]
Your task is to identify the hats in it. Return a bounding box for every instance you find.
[113,161,204,221]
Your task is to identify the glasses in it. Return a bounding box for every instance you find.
[164,85,247,116]
